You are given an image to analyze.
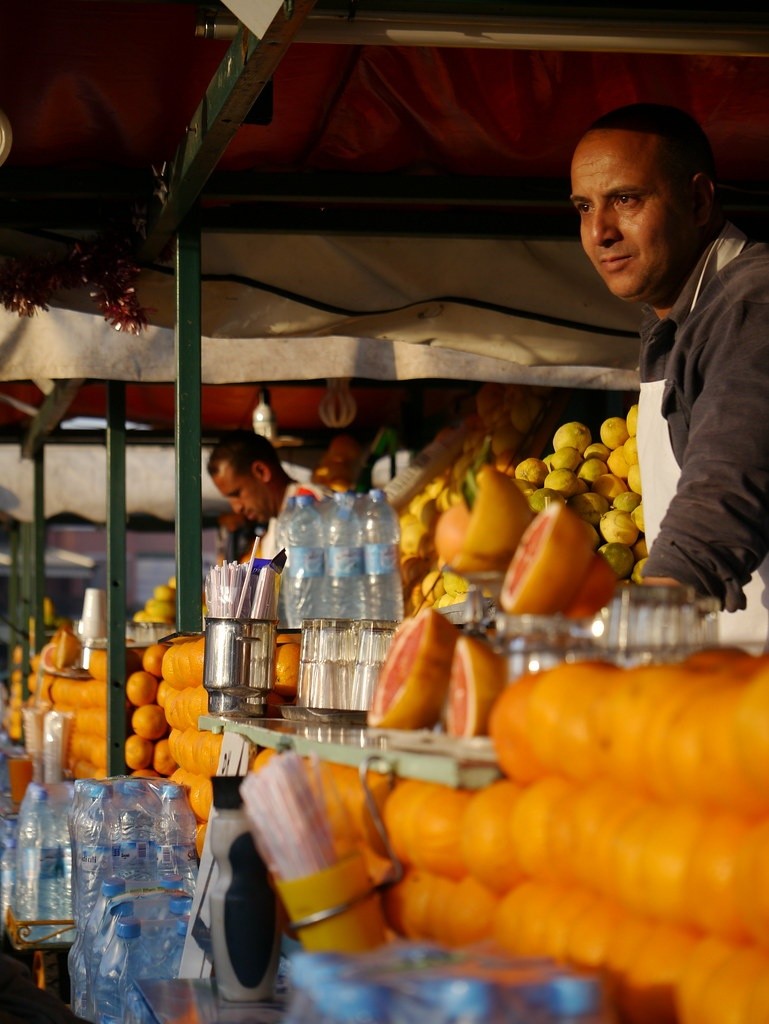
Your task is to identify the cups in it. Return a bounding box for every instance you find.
[296,618,402,712]
[127,619,171,643]
[203,617,279,718]
[21,706,71,785]
[504,584,720,684]
[276,852,388,955]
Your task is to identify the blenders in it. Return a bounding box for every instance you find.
[78,585,112,672]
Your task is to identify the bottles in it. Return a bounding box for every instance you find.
[0,732,200,1024]
[209,776,284,1004]
[282,940,603,1024]
[274,488,406,626]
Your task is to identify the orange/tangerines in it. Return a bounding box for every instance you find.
[12,382,769,1024]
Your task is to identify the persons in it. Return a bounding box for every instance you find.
[570,100,769,612]
[208,431,326,524]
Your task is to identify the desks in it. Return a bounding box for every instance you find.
[7,716,507,1024]
